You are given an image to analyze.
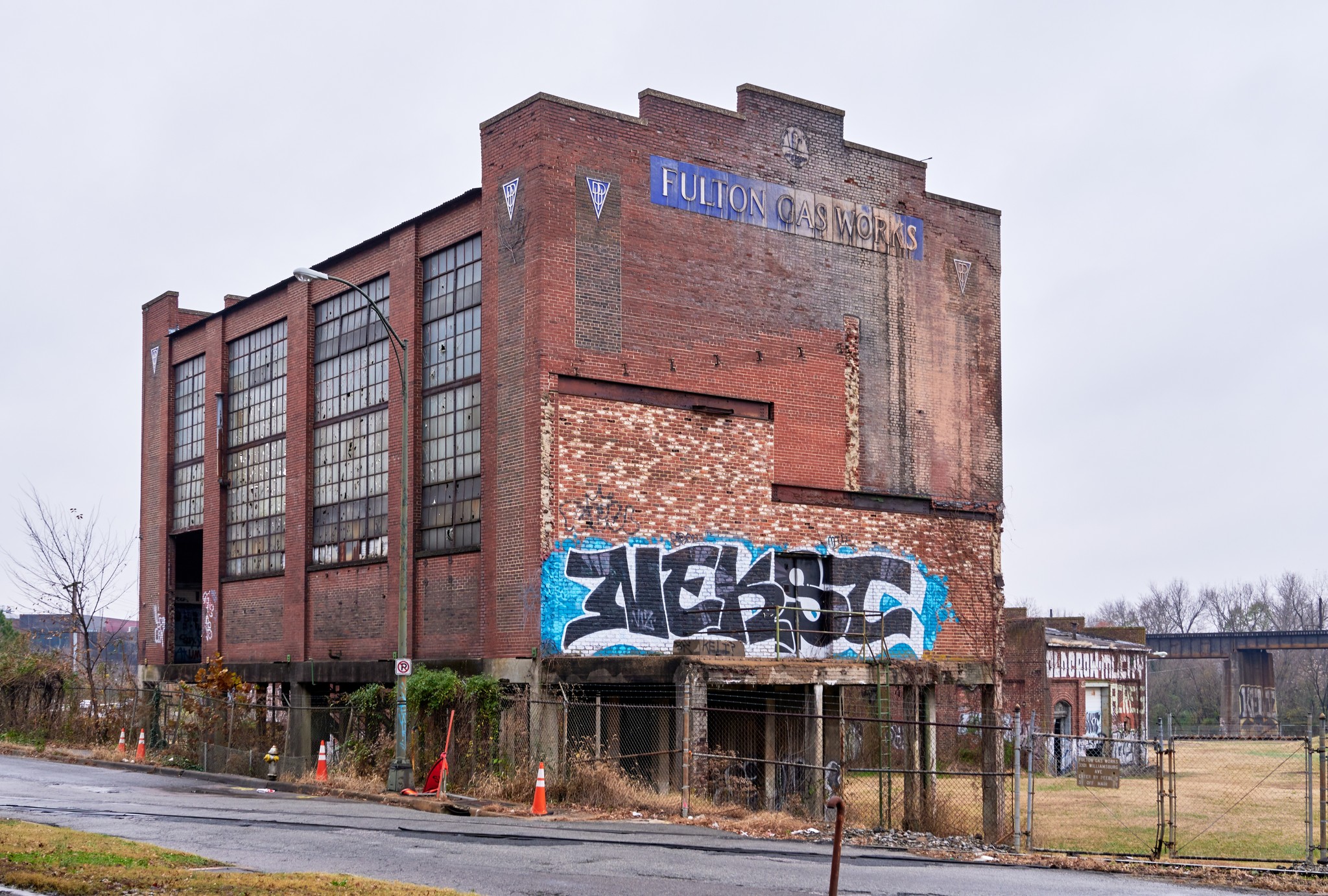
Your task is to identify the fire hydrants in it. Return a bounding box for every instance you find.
[263,745,279,781]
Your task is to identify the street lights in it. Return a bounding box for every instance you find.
[292,265,417,791]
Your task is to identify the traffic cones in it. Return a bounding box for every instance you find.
[136,728,145,759]
[117,728,126,752]
[315,740,328,780]
[530,761,549,815]
